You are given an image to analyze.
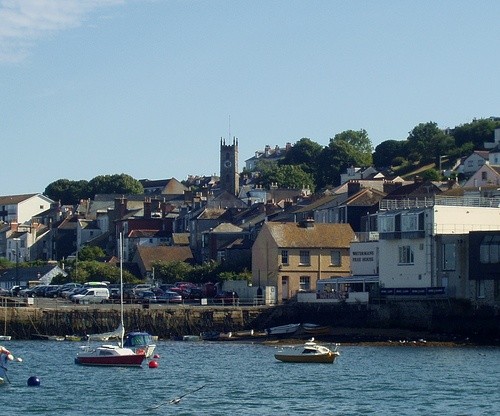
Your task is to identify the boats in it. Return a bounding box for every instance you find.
[0,347,12,384]
[119,331,157,357]
[274,336,340,364]
[32,331,118,341]
[0,335,12,341]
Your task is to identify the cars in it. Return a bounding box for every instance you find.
[0,282,216,304]
[215,291,238,303]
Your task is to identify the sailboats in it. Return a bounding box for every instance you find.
[75,232,145,368]
